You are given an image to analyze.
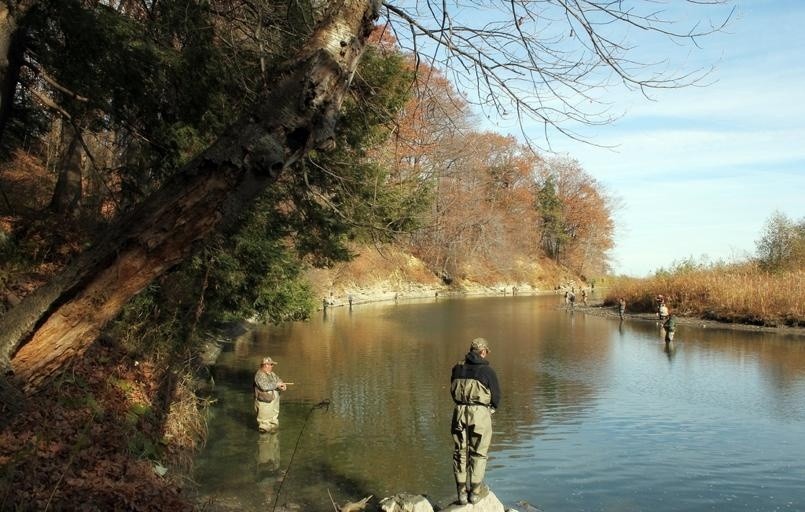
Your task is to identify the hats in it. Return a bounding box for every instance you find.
[261,357,278,365]
[471,338,489,353]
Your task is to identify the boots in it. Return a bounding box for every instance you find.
[457,483,469,505]
[470,481,489,503]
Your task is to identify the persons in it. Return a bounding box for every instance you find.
[253,357,286,433]
[655,294,664,316]
[580,288,588,306]
[659,304,669,319]
[451,337,501,504]
[565,291,575,306]
[619,298,626,321]
[663,313,676,341]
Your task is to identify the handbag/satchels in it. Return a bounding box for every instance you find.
[258,391,273,402]
